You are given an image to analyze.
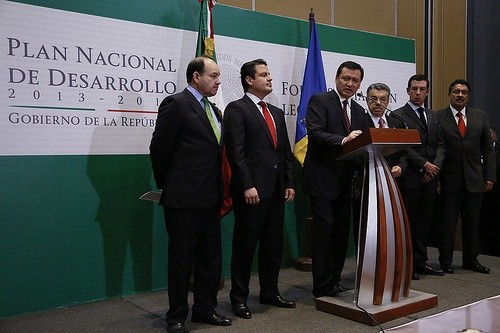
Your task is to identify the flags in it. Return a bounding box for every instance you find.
[196,0,217,63]
[293,17,327,167]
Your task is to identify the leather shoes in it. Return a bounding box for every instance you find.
[412,271,419,280]
[440,263,454,274]
[462,258,491,274]
[259,295,296,308]
[169,320,190,333]
[415,263,445,276]
[192,305,232,325]
[231,302,251,319]
[314,284,351,300]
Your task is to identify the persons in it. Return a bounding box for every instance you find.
[222,58,297,319]
[150,57,232,333]
[354,83,408,250]
[301,60,373,297]
[390,74,446,279]
[437,79,495,274]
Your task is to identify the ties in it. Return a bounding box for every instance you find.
[257,102,276,147]
[456,112,465,136]
[201,96,220,145]
[417,107,427,129]
[378,118,386,128]
[342,100,351,131]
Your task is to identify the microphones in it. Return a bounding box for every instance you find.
[357,92,408,130]
